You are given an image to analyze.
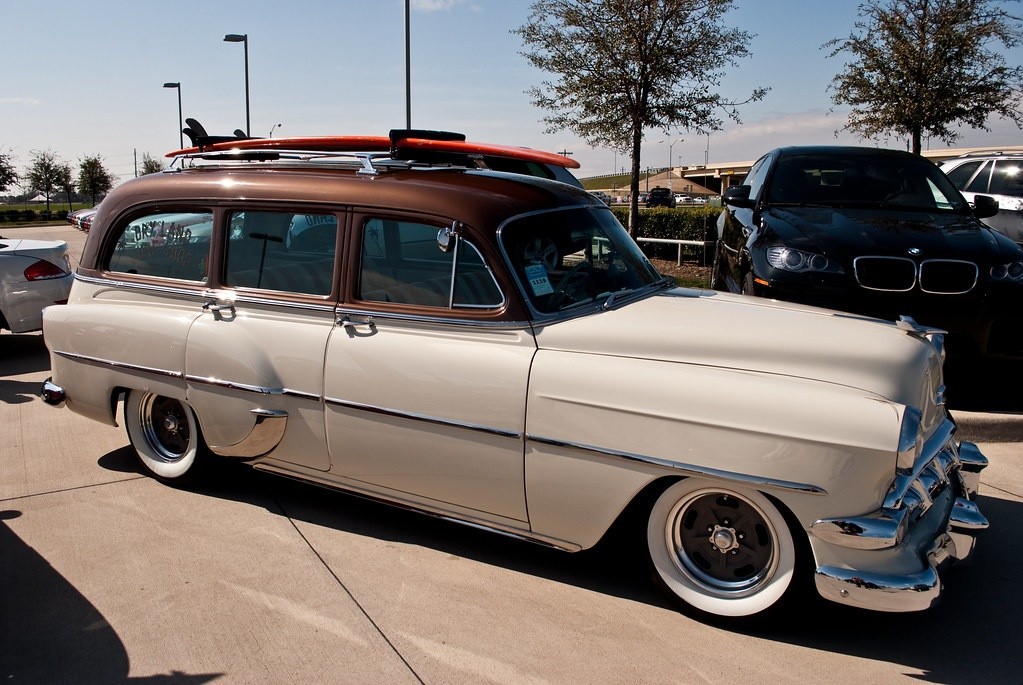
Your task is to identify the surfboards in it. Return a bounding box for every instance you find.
[166,133,583,172]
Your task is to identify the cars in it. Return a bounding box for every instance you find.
[1,237,74,335]
[693,197,709,204]
[66,201,477,263]
[39,127,990,625]
[675,193,692,203]
[646,187,676,208]
[709,146,1023,370]
[590,191,646,205]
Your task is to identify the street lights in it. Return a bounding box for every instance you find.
[270,123,281,138]
[223,33,250,142]
[162,82,185,171]
[658,139,684,188]
[696,127,709,164]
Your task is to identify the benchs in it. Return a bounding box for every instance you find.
[593,237,716,267]
[232,256,334,294]
[361,269,499,307]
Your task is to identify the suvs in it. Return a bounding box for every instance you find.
[925,150,1022,251]
